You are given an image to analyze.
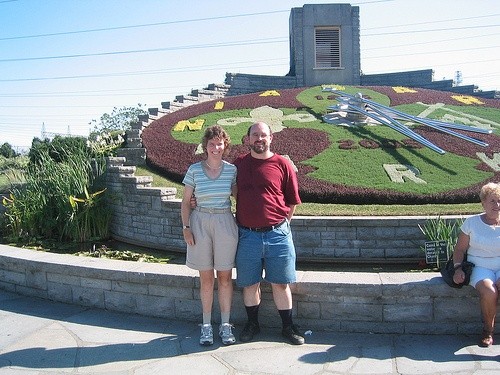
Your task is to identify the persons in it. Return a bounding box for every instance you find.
[191,121,304,345]
[181,125,237,346]
[453,181,500,347]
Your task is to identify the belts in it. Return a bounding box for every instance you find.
[239,219,287,233]
[195,206,231,215]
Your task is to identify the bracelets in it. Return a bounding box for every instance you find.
[453,263,462,268]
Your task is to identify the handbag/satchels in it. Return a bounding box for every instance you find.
[440,253,474,289]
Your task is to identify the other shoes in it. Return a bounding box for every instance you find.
[480,334,494,347]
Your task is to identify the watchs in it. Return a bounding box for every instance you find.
[182,226,189,229]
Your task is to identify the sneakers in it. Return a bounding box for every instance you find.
[200,324,214,345]
[281,325,306,344]
[218,323,237,346]
[239,322,259,342]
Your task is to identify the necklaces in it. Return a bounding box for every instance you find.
[206,160,223,169]
